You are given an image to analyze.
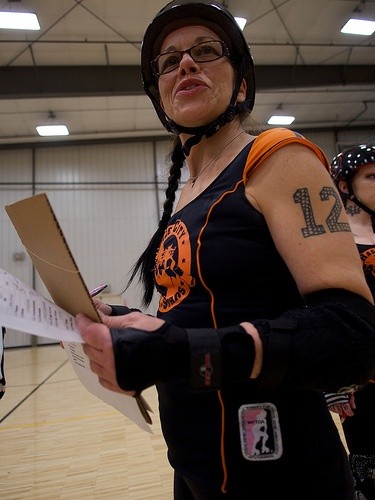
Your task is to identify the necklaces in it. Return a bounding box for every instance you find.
[188,130,245,187]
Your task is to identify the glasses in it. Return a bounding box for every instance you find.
[149,39,238,83]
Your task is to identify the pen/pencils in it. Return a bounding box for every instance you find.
[89,284,107,297]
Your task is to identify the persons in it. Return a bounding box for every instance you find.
[77,0,375,500]
[328,145,375,300]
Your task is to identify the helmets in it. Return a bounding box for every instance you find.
[329,144,375,188]
[141,0,256,124]
